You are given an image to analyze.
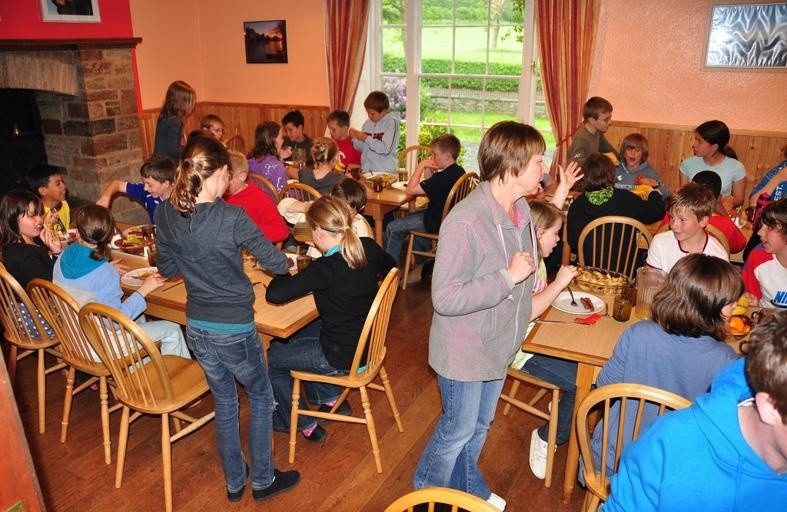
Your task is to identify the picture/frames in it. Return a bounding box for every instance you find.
[699,2,786,70]
[243,18,290,64]
[37,0,102,24]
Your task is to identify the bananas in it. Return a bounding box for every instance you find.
[730,297,750,315]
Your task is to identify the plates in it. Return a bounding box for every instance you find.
[551,291,606,316]
[390,181,410,190]
[108,219,157,260]
[728,218,745,229]
[119,267,159,287]
[284,250,300,270]
[362,171,386,177]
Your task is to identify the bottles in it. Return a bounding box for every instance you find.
[750,193,772,229]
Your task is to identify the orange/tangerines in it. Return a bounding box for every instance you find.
[727,316,751,334]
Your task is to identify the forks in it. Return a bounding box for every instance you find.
[568,286,576,307]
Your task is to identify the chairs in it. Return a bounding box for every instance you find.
[575,380,698,512]
[399,170,480,289]
[77,300,236,512]
[381,486,503,512]
[489,357,567,489]
[398,144,434,169]
[576,213,655,295]
[282,264,403,476]
[25,274,159,468]
[248,171,278,202]
[279,180,322,202]
[1,266,70,436]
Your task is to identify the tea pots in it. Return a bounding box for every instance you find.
[292,147,306,167]
[636,268,670,322]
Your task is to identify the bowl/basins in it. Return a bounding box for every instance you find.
[568,264,628,297]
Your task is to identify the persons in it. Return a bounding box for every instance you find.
[409,97,787,511]
[153,130,302,503]
[2,79,467,448]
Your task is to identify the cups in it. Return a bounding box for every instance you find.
[372,178,383,192]
[399,168,408,180]
[58,229,79,250]
[611,294,632,322]
[624,287,636,307]
[296,255,311,272]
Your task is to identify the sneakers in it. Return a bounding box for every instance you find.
[227,462,249,501]
[301,422,325,441]
[313,400,351,424]
[529,429,558,480]
[486,492,507,512]
[253,469,300,501]
